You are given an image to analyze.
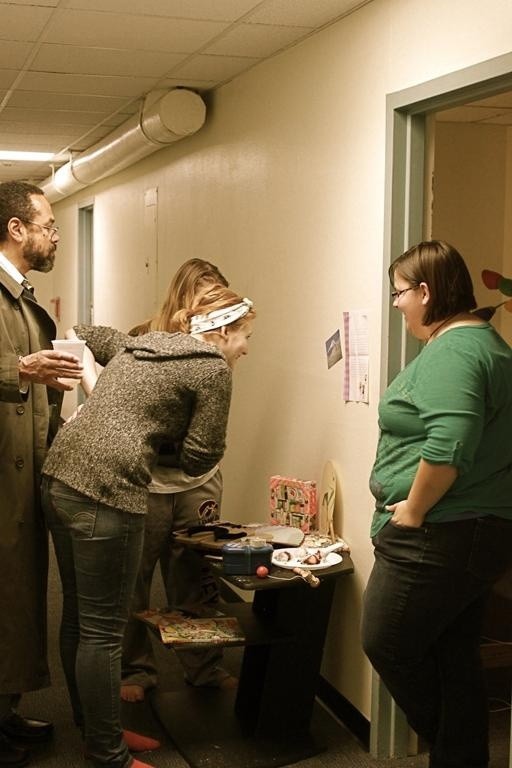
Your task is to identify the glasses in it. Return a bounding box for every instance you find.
[14,214,61,237]
[386,282,420,304]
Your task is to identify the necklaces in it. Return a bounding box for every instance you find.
[426,311,461,341]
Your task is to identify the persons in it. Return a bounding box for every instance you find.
[0,178,81,754]
[119,255,241,704]
[356,240,512,766]
[39,284,259,768]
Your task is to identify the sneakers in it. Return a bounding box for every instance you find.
[78,724,164,768]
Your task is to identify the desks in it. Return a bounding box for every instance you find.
[145,524,355,768]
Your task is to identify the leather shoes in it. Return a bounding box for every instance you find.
[0,705,53,767]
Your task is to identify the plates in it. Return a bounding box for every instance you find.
[271,547,344,572]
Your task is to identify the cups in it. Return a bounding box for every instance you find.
[51,339,87,388]
